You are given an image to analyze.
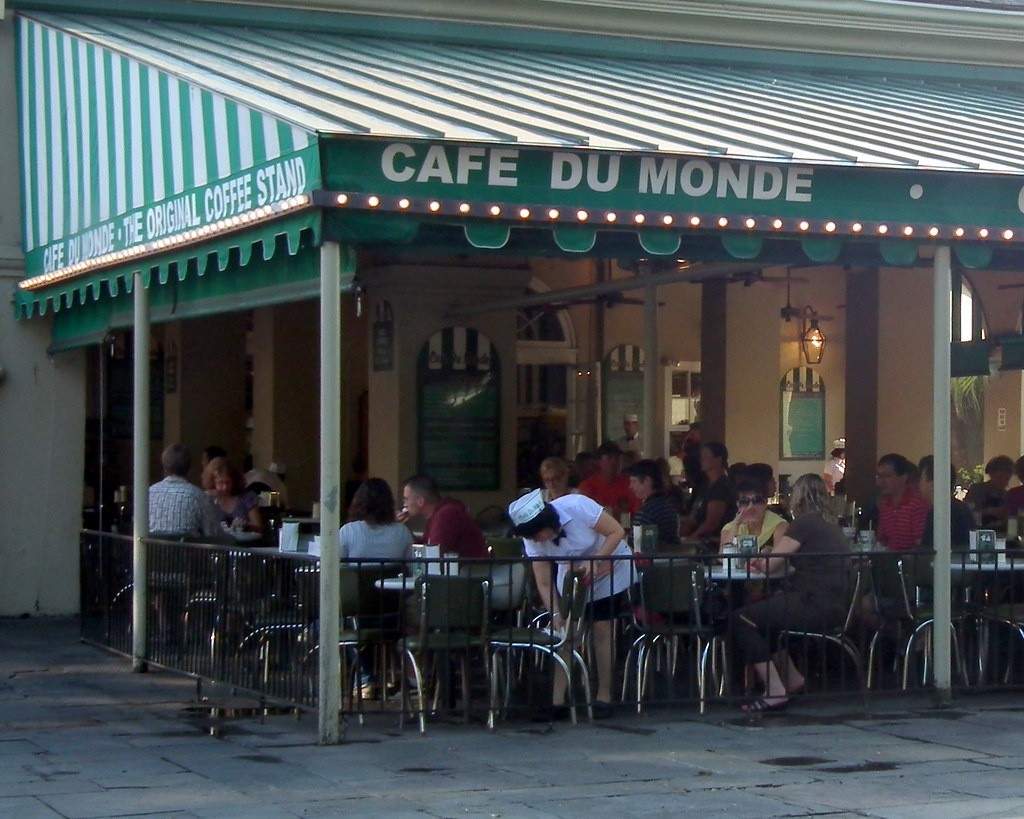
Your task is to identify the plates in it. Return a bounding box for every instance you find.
[233,532,260,541]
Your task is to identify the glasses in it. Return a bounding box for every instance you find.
[875,474,897,479]
[542,474,563,485]
[738,496,764,505]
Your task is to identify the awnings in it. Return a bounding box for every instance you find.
[6,2,1024,750]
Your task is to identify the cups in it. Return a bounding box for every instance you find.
[842,527,857,540]
[279,528,300,552]
[859,530,875,551]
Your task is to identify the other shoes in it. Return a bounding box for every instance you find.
[353,682,374,699]
[534,704,570,723]
[377,680,426,699]
[594,697,615,717]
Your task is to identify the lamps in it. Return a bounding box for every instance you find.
[801,306,826,364]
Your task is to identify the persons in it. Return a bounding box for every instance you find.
[146,440,225,624]
[391,475,493,688]
[195,444,290,644]
[509,413,790,720]
[860,453,977,618]
[962,455,1024,532]
[823,437,846,497]
[732,473,850,709]
[338,477,416,701]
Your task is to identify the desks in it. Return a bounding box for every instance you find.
[232,530,258,541]
[851,544,888,552]
[281,518,320,524]
[931,551,1024,680]
[374,577,485,723]
[700,564,796,579]
[257,543,313,674]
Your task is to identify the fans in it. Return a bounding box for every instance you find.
[688,267,808,288]
[550,256,666,310]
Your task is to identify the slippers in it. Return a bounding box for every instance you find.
[742,699,786,712]
[789,682,810,700]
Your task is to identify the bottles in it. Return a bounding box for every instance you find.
[722,542,736,570]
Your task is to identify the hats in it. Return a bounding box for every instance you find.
[625,413,638,422]
[508,488,545,527]
[599,443,623,455]
[835,439,846,449]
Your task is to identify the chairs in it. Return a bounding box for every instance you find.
[106,516,1024,734]
